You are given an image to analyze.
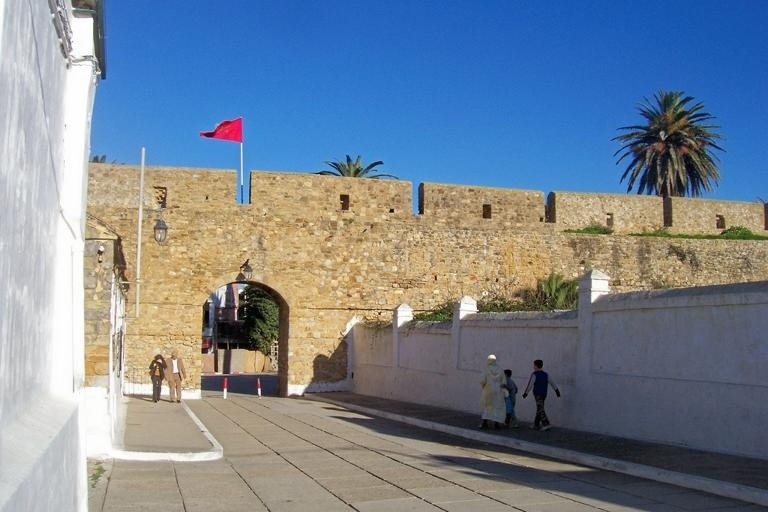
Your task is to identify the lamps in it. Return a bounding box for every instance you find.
[239,259,253,280]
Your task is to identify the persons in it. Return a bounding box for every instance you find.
[522,360,560,431]
[149,350,186,402]
[477,353,518,428]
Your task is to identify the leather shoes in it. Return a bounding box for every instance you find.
[154,398,180,403]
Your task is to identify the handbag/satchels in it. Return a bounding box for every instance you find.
[149,370,155,376]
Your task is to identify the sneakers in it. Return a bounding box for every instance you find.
[479,424,521,432]
[529,423,553,432]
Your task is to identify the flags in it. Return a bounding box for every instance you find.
[200,117,242,142]
